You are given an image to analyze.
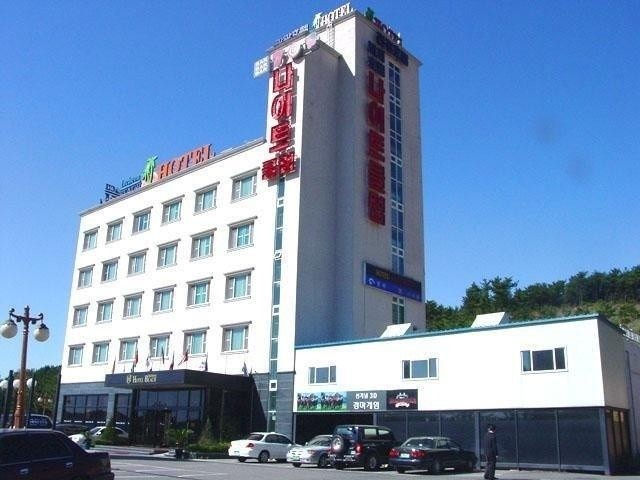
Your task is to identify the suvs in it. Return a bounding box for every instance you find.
[328,423,401,471]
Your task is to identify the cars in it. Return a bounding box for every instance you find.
[0,429,115,480]
[228,431,303,462]
[286,434,333,469]
[68,425,129,448]
[388,435,477,475]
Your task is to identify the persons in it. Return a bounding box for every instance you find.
[484,425,498,479]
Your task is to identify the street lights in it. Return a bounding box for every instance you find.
[1,369,21,426]
[1,305,50,427]
[25,373,38,428]
[37,393,54,416]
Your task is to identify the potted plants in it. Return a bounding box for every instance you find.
[165,428,194,459]
[83,431,92,449]
